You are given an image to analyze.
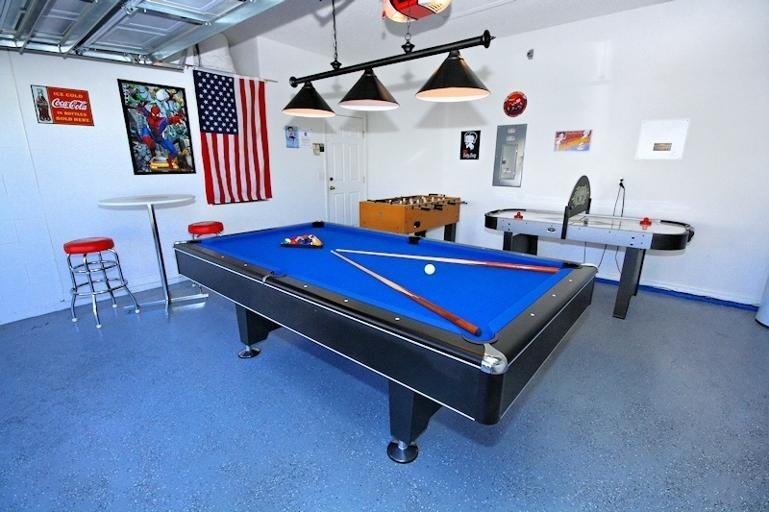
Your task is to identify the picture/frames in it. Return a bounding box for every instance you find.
[116,78,196,175]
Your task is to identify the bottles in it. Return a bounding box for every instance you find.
[499,127,519,180]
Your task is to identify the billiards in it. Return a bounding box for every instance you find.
[284,234,322,246]
[425,263,435,276]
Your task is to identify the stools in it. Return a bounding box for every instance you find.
[63,236,141,330]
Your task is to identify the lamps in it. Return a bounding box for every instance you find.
[279,0,496,119]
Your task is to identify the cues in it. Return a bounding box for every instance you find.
[336,248,559,273]
[330,249,482,337]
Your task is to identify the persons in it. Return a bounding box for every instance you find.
[138,102,182,171]
[287,126,297,147]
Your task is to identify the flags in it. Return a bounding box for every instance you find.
[192,69,273,206]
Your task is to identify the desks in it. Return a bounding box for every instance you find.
[483,174,696,320]
